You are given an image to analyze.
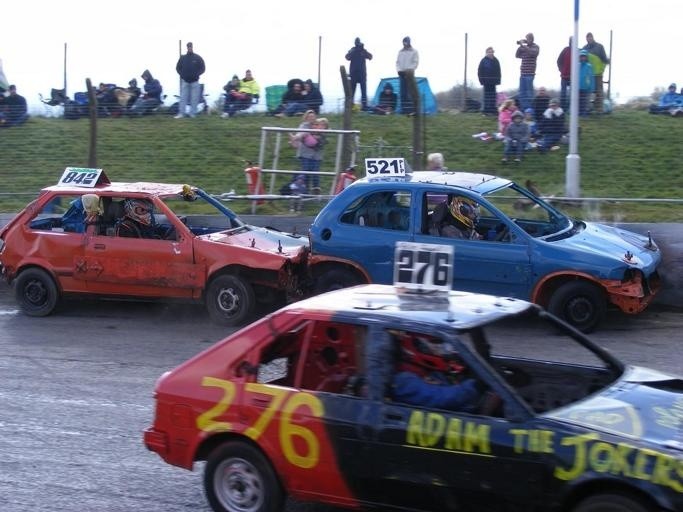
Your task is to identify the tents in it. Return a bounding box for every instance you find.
[371,77,436,114]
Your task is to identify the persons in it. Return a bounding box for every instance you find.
[557,33,610,114]
[271,78,324,118]
[288,110,329,193]
[650,83,682,117]
[515,33,540,107]
[114,198,168,240]
[345,37,373,110]
[372,82,398,114]
[426,152,444,171]
[176,42,205,119]
[94,70,162,115]
[478,46,502,112]
[222,70,260,117]
[498,88,566,162]
[1,84,27,127]
[396,36,419,114]
[388,331,513,410]
[439,195,483,240]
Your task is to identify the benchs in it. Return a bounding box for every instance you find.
[217,94,258,115]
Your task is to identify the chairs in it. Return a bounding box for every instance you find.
[430,202,449,237]
[106,201,128,236]
[139,94,167,112]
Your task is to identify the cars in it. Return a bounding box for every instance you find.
[143,278,682,511]
[307,157,661,333]
[0,167,310,325]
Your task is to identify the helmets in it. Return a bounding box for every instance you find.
[400,331,465,375]
[123,197,154,227]
[445,192,480,229]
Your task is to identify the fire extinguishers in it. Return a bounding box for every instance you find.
[241,159,266,205]
[336,165,359,194]
